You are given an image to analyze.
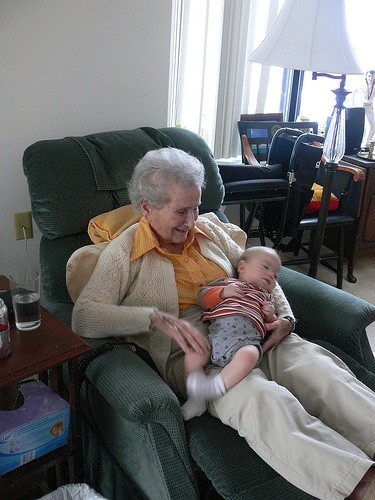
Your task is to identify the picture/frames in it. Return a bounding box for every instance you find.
[241,113,283,163]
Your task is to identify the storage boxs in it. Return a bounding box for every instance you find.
[0,379,70,475]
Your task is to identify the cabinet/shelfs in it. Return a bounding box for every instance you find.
[315,152,375,258]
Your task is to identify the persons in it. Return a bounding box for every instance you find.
[352,70,375,152]
[71,145,375,500]
[177,246,283,422]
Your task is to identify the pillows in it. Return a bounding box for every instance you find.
[64,212,221,306]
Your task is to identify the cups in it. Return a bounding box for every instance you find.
[9,269,42,331]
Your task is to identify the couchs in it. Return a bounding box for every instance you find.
[22,127,374,500]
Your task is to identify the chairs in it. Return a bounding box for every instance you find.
[238,121,365,284]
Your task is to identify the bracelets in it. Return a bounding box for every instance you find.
[284,316,295,335]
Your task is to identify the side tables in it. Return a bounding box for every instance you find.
[0,274,93,500]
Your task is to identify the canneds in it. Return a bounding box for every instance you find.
[0,298,11,359]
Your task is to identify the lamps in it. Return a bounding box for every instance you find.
[247,1,366,280]
